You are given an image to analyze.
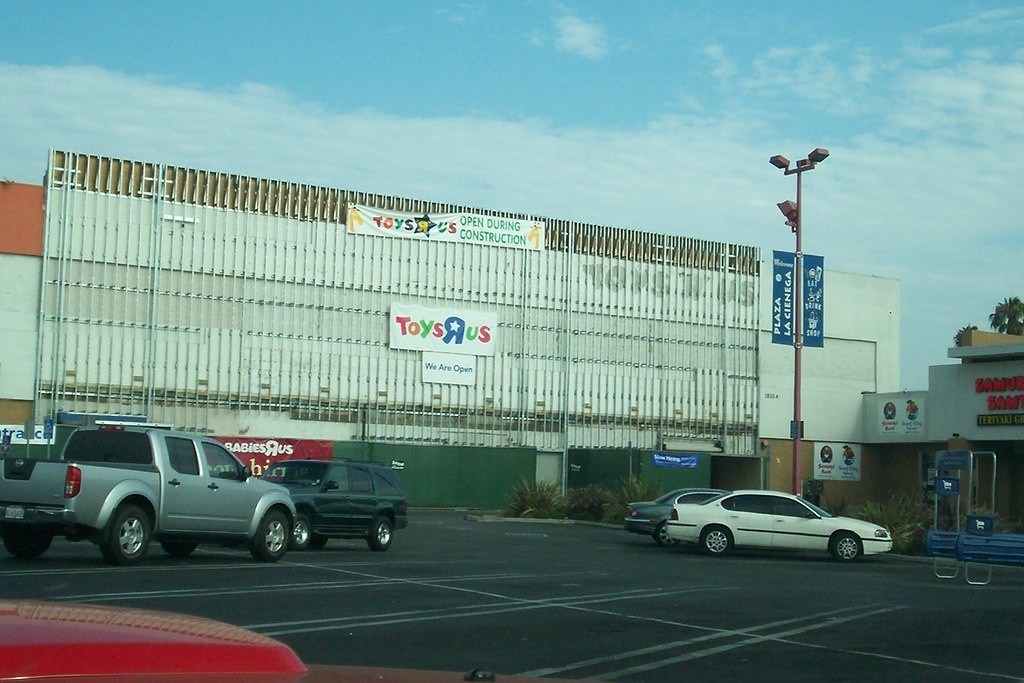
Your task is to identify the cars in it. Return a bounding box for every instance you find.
[0,598,560,683]
[665,489,893,562]
[623,488,731,548]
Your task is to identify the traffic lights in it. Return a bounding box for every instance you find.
[2,445,9,453]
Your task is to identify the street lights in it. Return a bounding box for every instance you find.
[770,147,828,497]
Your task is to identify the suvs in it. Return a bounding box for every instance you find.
[252,458,409,551]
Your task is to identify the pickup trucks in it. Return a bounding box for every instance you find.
[0,425,296,566]
